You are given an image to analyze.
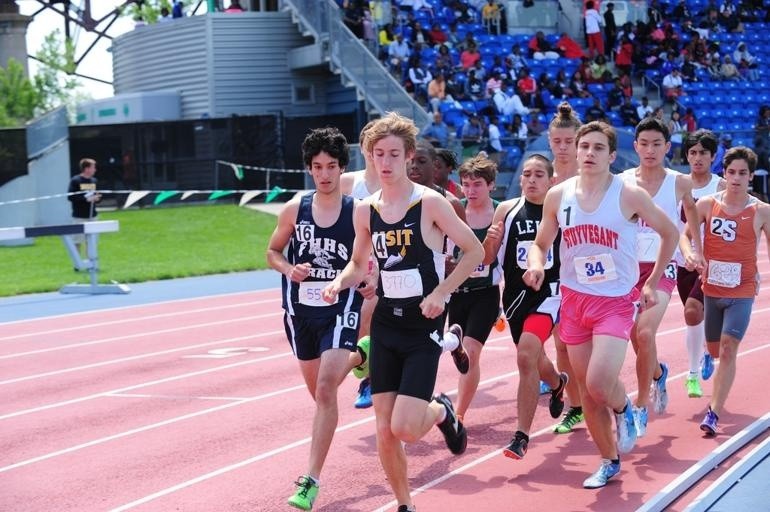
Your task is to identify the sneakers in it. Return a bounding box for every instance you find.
[504,431,529,460]
[288,475,319,510]
[612,362,667,454]
[540,372,584,432]
[687,353,714,397]
[353,336,373,408]
[431,393,467,454]
[449,324,469,373]
[583,456,621,487]
[700,412,718,435]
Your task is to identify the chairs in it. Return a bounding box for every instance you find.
[379,0,770,182]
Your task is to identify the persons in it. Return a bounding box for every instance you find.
[483,99,726,486]
[224,0,248,12]
[338,1,770,167]
[267,129,380,512]
[322,110,501,512]
[67,158,102,271]
[678,146,770,439]
[172,3,184,18]
[156,8,173,21]
[135,17,148,27]
